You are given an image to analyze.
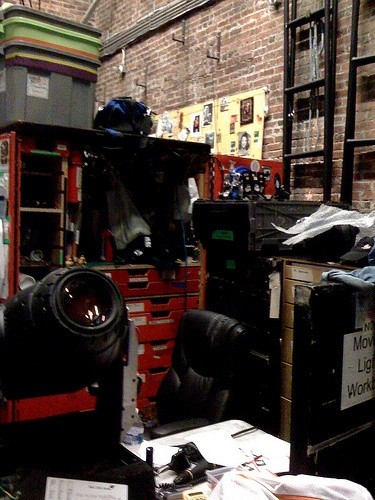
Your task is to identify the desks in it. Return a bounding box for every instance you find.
[124,419,373,500]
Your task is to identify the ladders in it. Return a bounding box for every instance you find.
[339,0,375,205]
[339,0,375,208]
[283,0,336,203]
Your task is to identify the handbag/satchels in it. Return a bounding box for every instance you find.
[93,96,153,136]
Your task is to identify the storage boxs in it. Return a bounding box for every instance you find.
[0,4,102,130]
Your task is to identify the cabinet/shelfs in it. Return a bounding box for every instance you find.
[0,120,207,426]
[195,156,373,468]
